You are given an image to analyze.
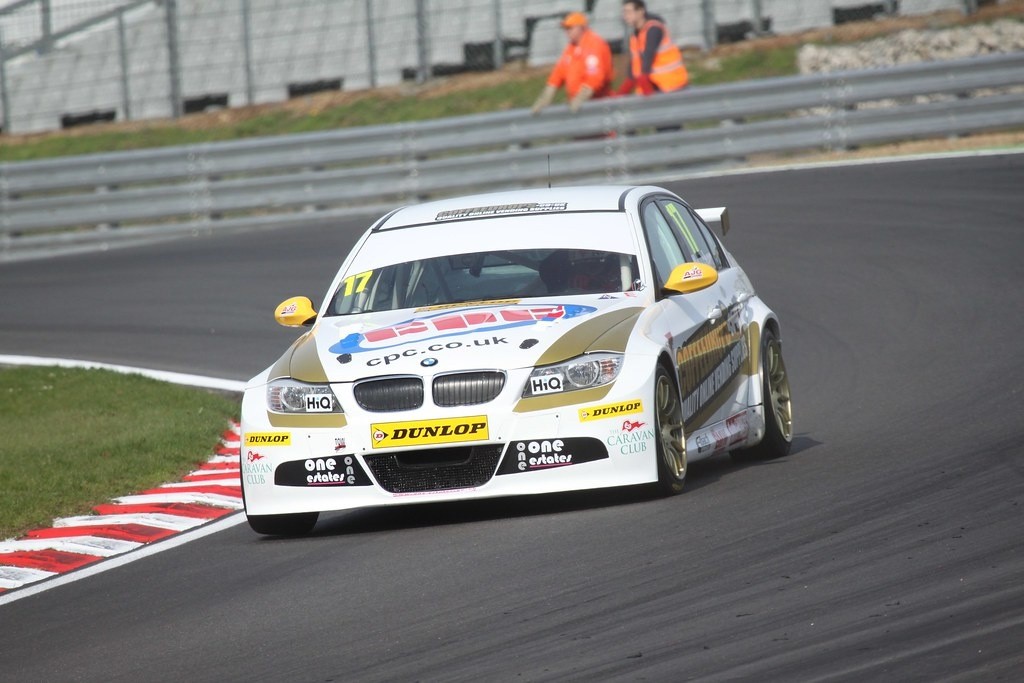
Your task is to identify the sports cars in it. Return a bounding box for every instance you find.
[237,182,794,538]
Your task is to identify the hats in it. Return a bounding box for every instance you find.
[561,12,589,28]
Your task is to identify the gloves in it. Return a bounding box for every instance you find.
[571,85,593,113]
[615,76,634,96]
[635,72,657,97]
[533,85,558,114]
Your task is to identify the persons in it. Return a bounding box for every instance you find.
[522,250,643,296]
[611,0,691,133]
[533,11,617,138]
